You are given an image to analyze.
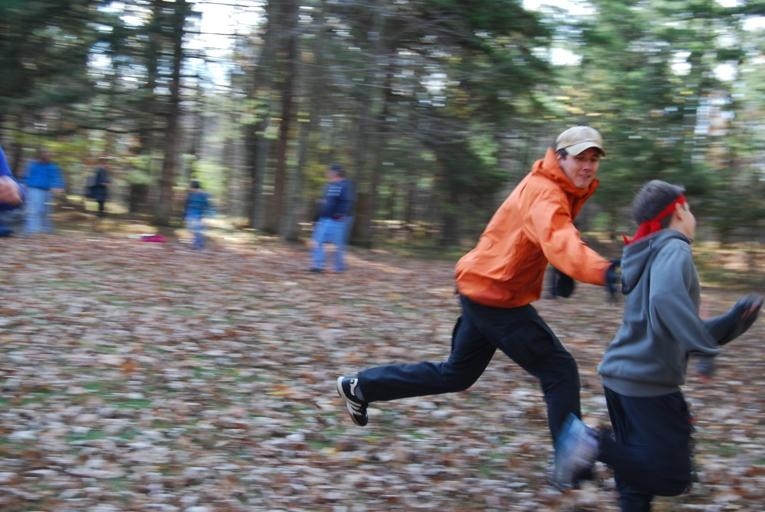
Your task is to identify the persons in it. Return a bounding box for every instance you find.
[334,126,620,499]
[89,157,113,212]
[308,163,361,274]
[20,149,65,233]
[183,181,213,251]
[0,143,24,235]
[555,179,765,512]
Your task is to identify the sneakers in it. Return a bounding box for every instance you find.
[547,413,602,494]
[336,376,369,427]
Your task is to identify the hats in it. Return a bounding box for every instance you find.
[556,126,606,158]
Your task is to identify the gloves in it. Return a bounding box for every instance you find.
[550,266,573,298]
[606,258,620,295]
